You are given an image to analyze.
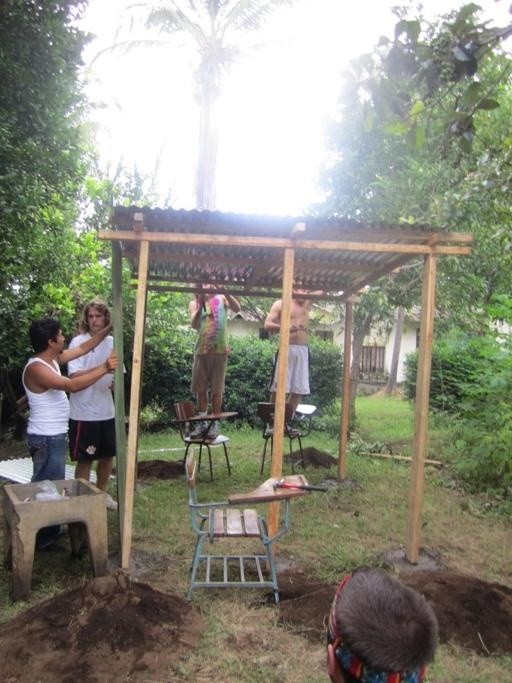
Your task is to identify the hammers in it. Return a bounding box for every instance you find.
[196,282,207,312]
[273,478,328,492]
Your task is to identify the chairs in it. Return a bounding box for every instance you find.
[184,445,312,606]
[172,400,239,481]
[257,399,316,475]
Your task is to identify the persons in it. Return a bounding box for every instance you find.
[264,286,325,436]
[318,564,441,683]
[21,317,120,548]
[63,301,128,493]
[187,277,242,440]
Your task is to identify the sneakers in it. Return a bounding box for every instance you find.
[265,423,273,436]
[189,423,219,440]
[105,493,118,511]
[284,422,300,436]
